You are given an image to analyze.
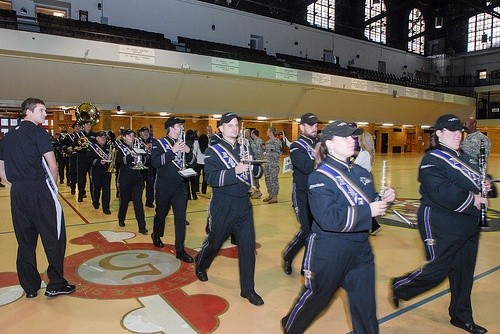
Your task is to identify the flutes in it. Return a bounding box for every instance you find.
[380,161,387,204]
[476,139,490,232]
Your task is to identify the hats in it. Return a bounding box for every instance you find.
[71,123,81,127]
[83,119,93,124]
[321,120,362,141]
[300,113,321,125]
[165,116,185,129]
[60,130,68,134]
[435,114,468,131]
[95,131,107,138]
[140,126,150,132]
[124,129,136,135]
[221,112,239,123]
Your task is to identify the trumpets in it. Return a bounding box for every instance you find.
[178,129,186,169]
[238,121,256,194]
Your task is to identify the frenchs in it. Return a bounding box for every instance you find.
[75,102,100,126]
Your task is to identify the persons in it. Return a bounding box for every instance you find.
[387,113,489,334]
[279,119,396,334]
[461,116,493,172]
[280,112,325,274]
[45,116,286,234]
[150,117,196,263]
[193,113,266,305]
[353,131,383,236]
[1,96,76,299]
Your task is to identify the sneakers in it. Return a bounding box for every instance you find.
[26,286,40,298]
[44,284,76,297]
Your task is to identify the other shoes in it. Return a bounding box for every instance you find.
[263,194,278,203]
[250,190,262,200]
[58,177,154,235]
[369,226,382,235]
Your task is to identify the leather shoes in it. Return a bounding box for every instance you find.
[447,317,487,334]
[184,220,189,225]
[150,232,164,247]
[390,276,400,309]
[281,250,292,275]
[194,256,209,281]
[239,291,264,306]
[175,249,194,263]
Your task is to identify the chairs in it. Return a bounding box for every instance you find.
[0,7,500,98]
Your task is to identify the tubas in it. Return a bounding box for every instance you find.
[100,145,115,173]
[131,139,149,170]
